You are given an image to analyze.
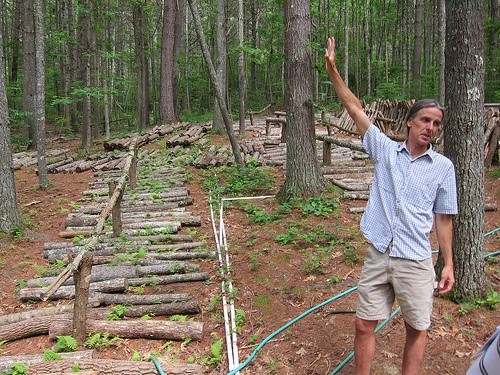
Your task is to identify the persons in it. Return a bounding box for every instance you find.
[324,35,458,374]
